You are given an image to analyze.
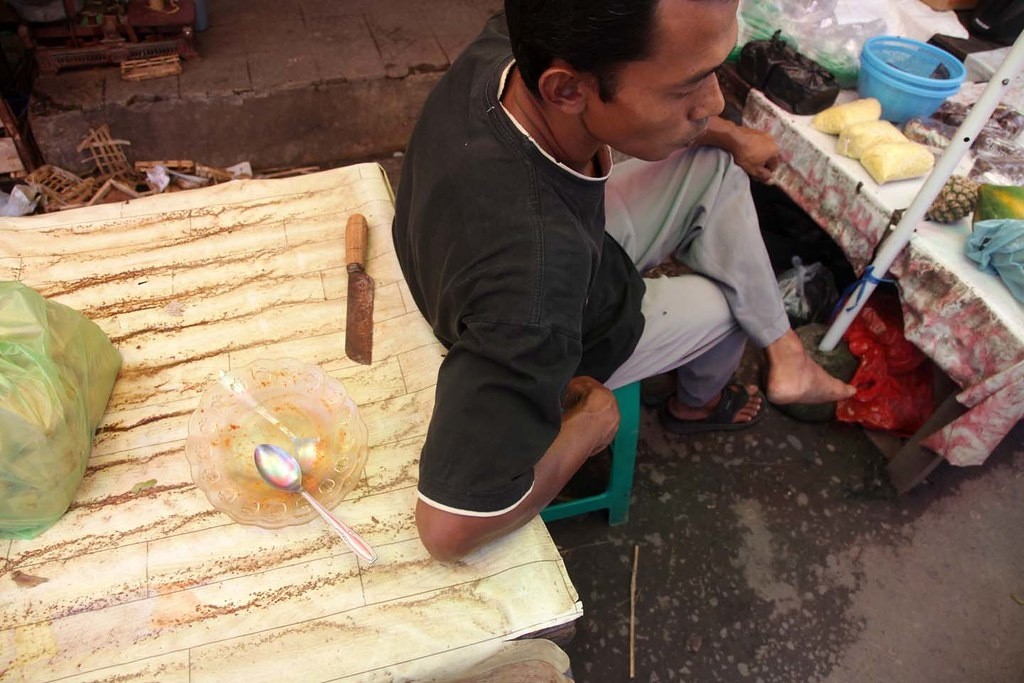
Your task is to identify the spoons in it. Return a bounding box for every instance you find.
[251,442,377,565]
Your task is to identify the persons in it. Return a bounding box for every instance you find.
[388,0,859,565]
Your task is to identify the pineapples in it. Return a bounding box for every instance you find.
[925,174,979,224]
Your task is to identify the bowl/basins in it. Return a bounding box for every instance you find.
[182,356,370,528]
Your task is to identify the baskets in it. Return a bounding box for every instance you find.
[857,35,966,123]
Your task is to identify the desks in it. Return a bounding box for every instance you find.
[738,76,1024,497]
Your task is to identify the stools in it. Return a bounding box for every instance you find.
[540,382,642,527]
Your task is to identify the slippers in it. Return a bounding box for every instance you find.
[658,382,768,434]
